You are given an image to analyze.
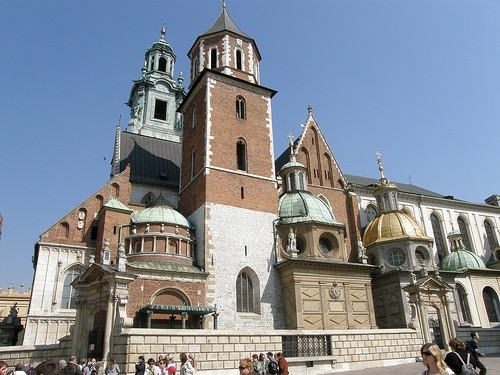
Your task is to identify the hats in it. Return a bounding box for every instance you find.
[59,360,67,366]
[168,366,176,373]
[267,352,273,356]
[139,356,144,360]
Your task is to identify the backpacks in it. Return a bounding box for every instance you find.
[455,351,480,375]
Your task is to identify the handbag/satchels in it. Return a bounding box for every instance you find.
[183,369,199,375]
[268,362,278,375]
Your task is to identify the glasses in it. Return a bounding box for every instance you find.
[421,352,432,356]
[253,357,257,358]
[159,362,162,364]
[239,366,245,369]
[149,363,154,365]
[2,368,5,370]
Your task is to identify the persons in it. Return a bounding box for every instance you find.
[465,331,487,375]
[239,358,261,375]
[288,227,297,250]
[420,343,456,375]
[179,353,195,375]
[135,356,145,375]
[188,355,197,372]
[251,352,289,375]
[444,337,476,375]
[105,357,120,375]
[358,236,364,256]
[0,355,105,375]
[146,353,177,375]
